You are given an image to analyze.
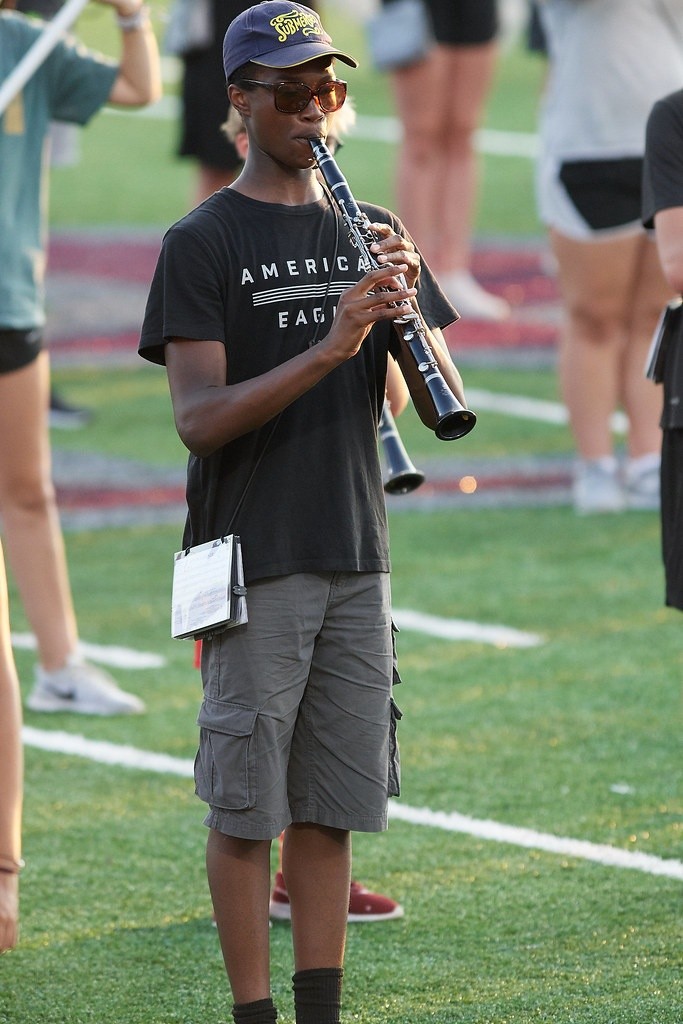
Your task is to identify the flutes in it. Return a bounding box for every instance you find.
[306,134,476,445]
[379,388,428,498]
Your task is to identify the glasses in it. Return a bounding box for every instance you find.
[236,77,347,113]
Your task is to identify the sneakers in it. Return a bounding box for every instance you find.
[24,657,143,715]
[269,874,407,922]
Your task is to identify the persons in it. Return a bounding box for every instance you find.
[138,3,467,1023]
[529,4,682,521]
[173,1,321,216]
[2,566,26,947]
[376,0,513,319]
[642,87,683,609]
[1,0,164,715]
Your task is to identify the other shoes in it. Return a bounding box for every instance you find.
[571,455,661,514]
[439,274,513,319]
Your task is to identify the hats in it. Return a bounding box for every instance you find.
[223,0,359,82]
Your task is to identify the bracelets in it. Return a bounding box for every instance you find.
[117,14,151,31]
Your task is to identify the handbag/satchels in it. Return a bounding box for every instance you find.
[171,533,247,638]
